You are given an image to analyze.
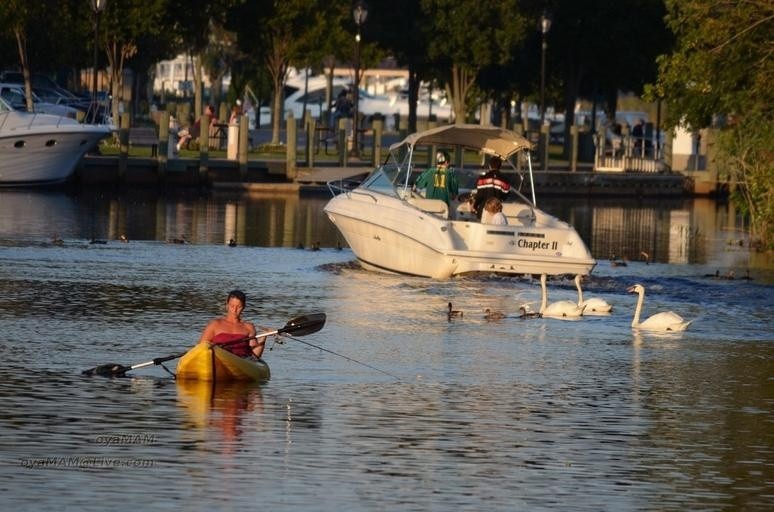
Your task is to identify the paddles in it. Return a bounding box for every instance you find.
[83,312,326,375]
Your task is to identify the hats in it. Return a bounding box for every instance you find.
[436,151,451,162]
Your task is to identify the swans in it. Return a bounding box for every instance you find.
[625,283,692,333]
[573,271,614,315]
[537,273,588,320]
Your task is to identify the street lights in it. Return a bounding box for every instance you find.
[88,0,108,121]
[349,2,369,156]
[538,7,553,125]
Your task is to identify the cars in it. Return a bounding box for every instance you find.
[0,69,112,123]
[513,108,655,154]
[245,80,400,134]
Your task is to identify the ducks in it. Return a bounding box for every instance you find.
[88,235,107,244]
[310,239,322,252]
[119,231,130,244]
[518,307,543,320]
[51,234,62,244]
[334,240,345,253]
[484,308,507,319]
[612,255,627,266]
[168,233,186,242]
[228,237,238,247]
[447,301,463,317]
[704,268,754,281]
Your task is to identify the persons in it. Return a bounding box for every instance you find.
[472,156,511,219]
[230,105,245,125]
[79,101,106,124]
[484,197,508,226]
[176,104,216,152]
[200,291,273,364]
[599,113,623,151]
[333,89,354,120]
[412,151,458,213]
[633,118,653,155]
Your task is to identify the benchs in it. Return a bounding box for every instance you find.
[127,125,162,157]
[183,120,255,153]
[316,124,368,158]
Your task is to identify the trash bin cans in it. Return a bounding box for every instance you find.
[227,116,240,161]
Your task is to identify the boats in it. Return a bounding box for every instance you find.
[0,93,116,185]
[174,380,261,433]
[323,121,602,285]
[175,340,271,384]
